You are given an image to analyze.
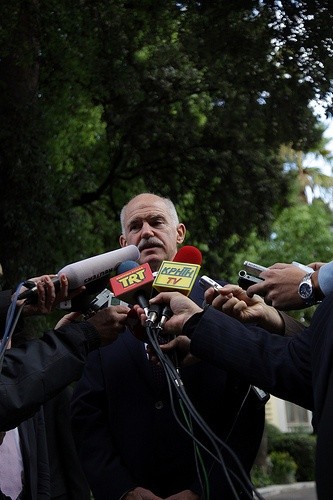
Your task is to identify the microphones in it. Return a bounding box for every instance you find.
[108,260,153,323]
[32,245,141,303]
[152,246,202,327]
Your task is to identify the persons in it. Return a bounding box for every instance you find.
[134,260,333,500]
[42,192,270,500]
[0,275,131,500]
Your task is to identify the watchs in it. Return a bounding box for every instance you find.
[298,273,322,305]
